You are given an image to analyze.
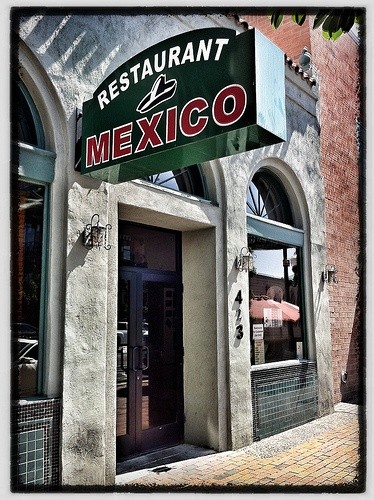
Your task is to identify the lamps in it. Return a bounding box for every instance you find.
[322,264,340,284]
[235,247,257,273]
[82,214,112,250]
